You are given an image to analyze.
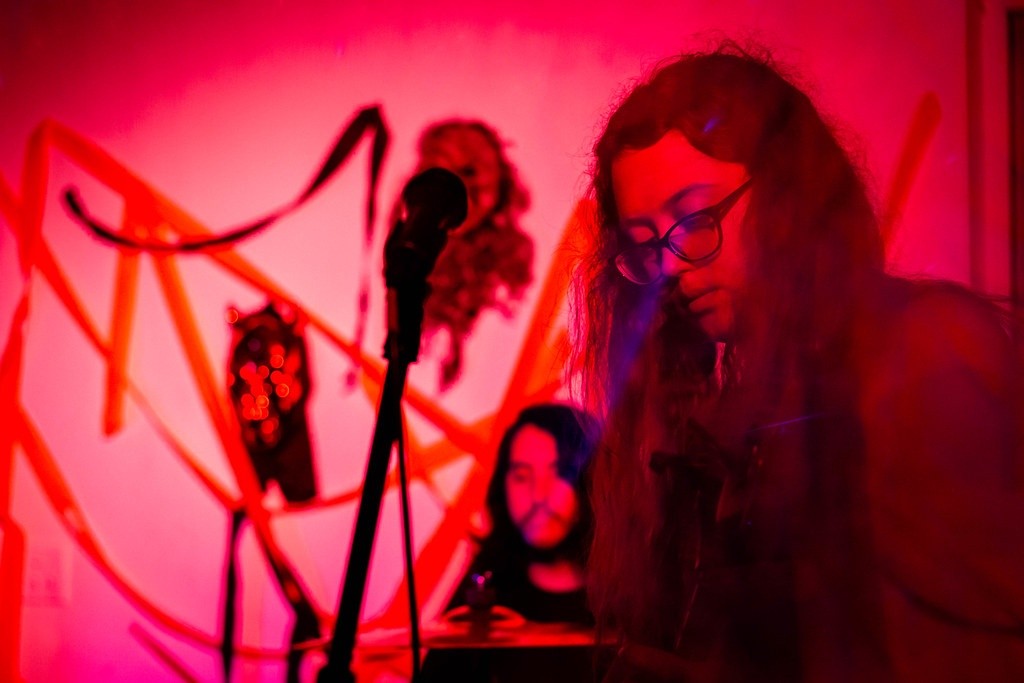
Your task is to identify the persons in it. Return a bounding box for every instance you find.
[566,52,1024,682]
[430,396,618,649]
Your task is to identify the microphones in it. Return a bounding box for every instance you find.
[383,166,468,288]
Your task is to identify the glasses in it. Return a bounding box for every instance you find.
[608,178,753,286]
